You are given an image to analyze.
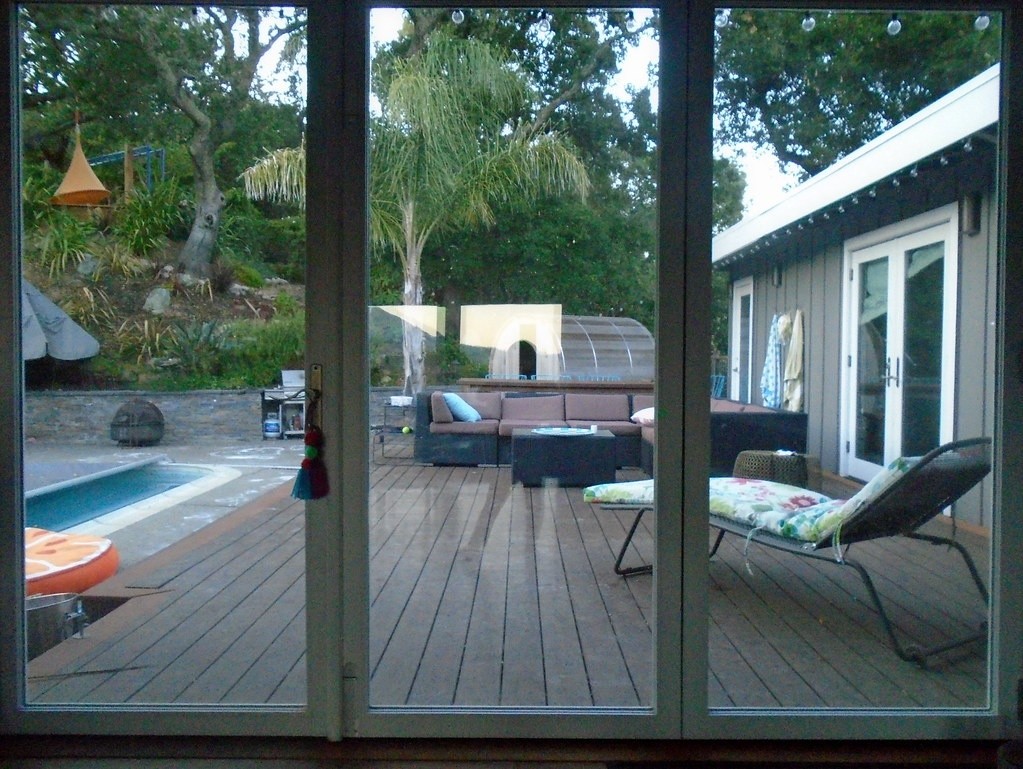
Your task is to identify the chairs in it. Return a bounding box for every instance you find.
[595,435,1002,660]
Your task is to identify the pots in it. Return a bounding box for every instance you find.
[27,593,88,660]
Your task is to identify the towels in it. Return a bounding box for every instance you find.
[760,309,805,414]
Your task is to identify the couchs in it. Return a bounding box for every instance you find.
[410,390,809,474]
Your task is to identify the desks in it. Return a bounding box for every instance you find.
[509,424,618,488]
